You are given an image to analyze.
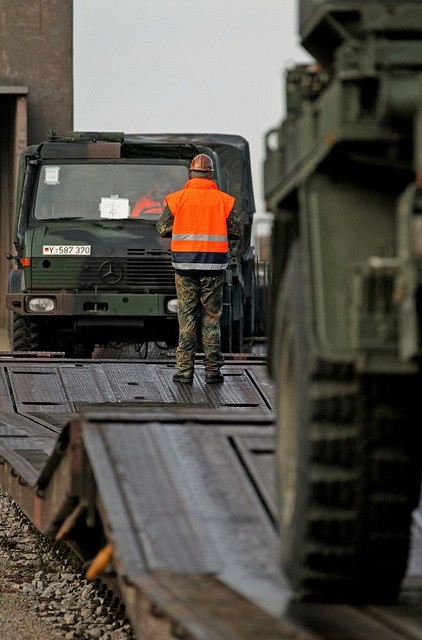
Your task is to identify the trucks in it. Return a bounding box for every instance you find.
[266,0,422,603]
[9,129,258,354]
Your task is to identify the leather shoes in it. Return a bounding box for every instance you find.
[172,370,193,383]
[205,369,224,383]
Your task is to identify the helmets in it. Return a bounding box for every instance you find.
[190,154,214,171]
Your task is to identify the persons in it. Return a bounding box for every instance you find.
[155,153,241,383]
[131,171,173,217]
